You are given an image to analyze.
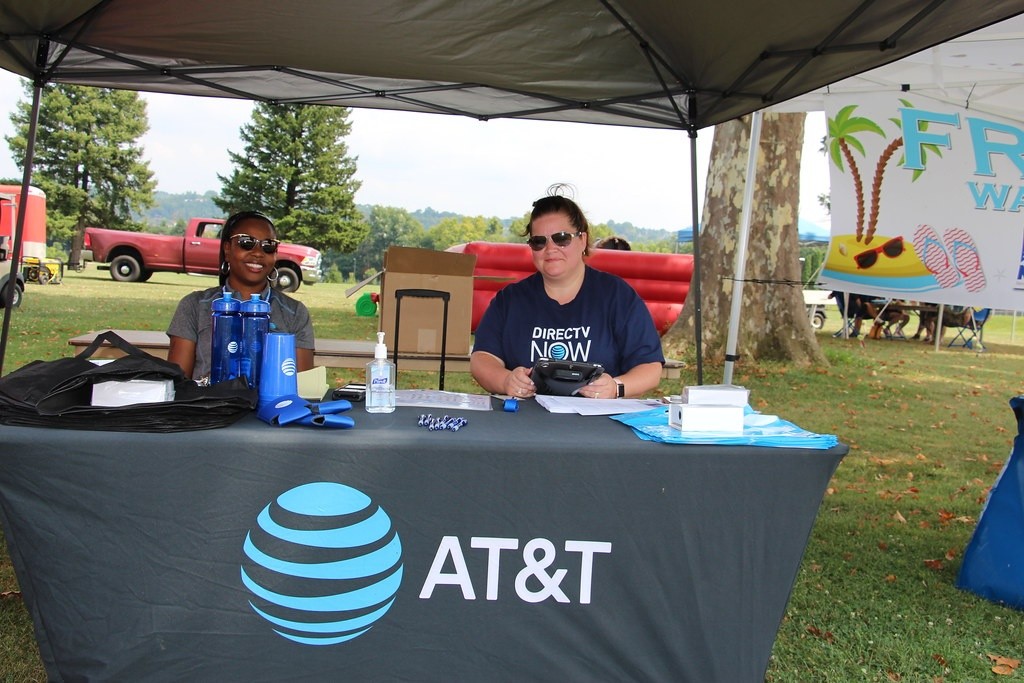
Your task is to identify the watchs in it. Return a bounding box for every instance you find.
[614,378,625,399]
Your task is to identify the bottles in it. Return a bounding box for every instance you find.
[211,291,270,391]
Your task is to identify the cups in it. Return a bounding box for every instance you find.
[259,331,298,415]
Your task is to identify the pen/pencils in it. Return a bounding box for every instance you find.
[417,413,469,433]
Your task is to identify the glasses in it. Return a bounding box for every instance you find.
[527,232,580,251]
[230,234,282,254]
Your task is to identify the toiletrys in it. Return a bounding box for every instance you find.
[364,332,396,414]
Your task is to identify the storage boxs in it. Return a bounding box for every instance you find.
[344,247,517,356]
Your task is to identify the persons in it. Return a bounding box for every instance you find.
[596,237,631,251]
[470,196,666,399]
[166,213,315,377]
[828,290,915,338]
[912,302,992,345]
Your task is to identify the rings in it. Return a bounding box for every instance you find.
[595,392,598,398]
[519,388,522,394]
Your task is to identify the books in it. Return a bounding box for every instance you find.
[297,366,329,402]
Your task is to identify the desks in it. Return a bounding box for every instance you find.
[0,391,850,683]
[873,301,938,342]
[68,329,686,380]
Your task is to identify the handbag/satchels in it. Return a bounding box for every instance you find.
[530,360,604,398]
[0,331,259,433]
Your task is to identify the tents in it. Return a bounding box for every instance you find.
[0,0,1024,387]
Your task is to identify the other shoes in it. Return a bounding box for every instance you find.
[923,336,930,342]
[911,333,919,339]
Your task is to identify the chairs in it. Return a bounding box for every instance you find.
[832,291,1005,349]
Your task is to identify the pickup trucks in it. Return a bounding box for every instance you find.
[81,218,323,295]
